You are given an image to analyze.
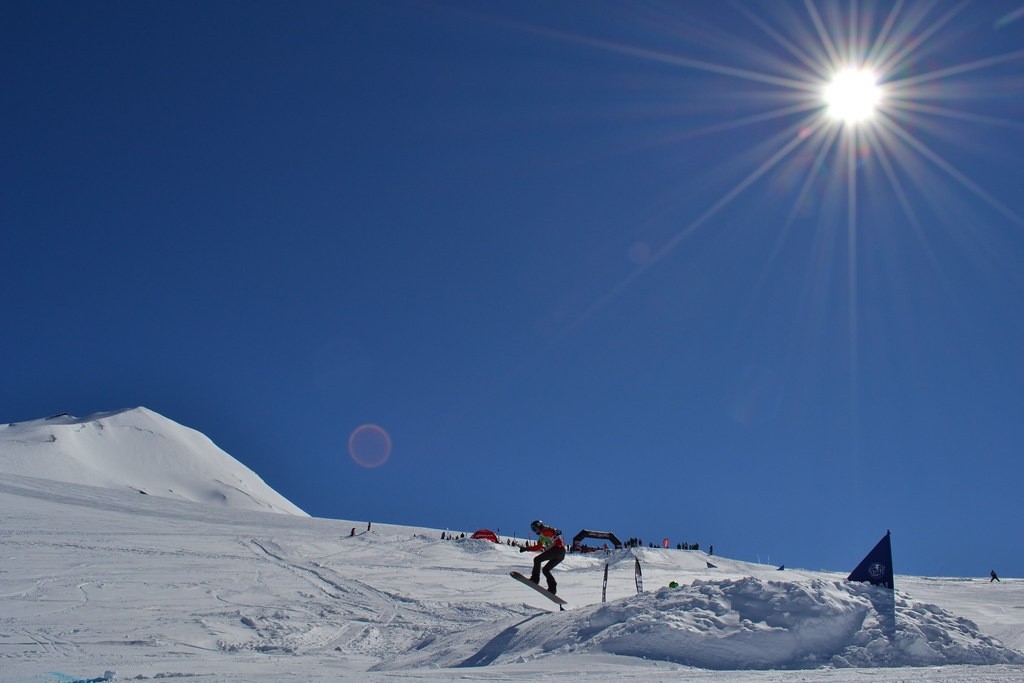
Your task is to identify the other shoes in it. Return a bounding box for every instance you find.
[529,576,539,585]
[547,585,556,595]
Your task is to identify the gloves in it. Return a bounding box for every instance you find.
[555,530,562,536]
[520,547,528,552]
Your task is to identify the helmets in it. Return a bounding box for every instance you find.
[531,521,543,535]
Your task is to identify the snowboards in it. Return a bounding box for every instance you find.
[509,571,566,604]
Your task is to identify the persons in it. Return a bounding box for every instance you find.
[368,521,371,531]
[351,528,355,536]
[709,544,713,555]
[624,537,700,550]
[506,539,612,555]
[990,570,1000,583]
[439,532,465,541]
[520,520,565,595]
